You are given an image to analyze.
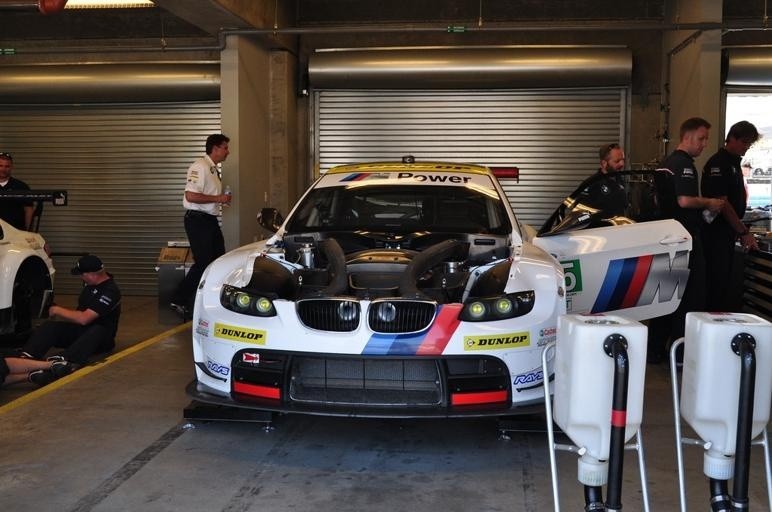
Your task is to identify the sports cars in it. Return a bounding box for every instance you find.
[0,189,68,336]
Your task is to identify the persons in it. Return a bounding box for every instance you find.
[701,122,758,311]
[576,143,626,229]
[655,117,725,367]
[0,152,33,231]
[170,134,232,315]
[0,357,81,387]
[18,256,121,365]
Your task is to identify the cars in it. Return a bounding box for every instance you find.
[184,156,692,443]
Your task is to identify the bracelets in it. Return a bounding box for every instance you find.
[740,228,749,236]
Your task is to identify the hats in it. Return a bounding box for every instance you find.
[71,255,103,274]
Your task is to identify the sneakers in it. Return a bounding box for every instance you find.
[26,355,75,384]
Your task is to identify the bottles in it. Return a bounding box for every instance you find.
[222,184,231,206]
[702,209,716,224]
